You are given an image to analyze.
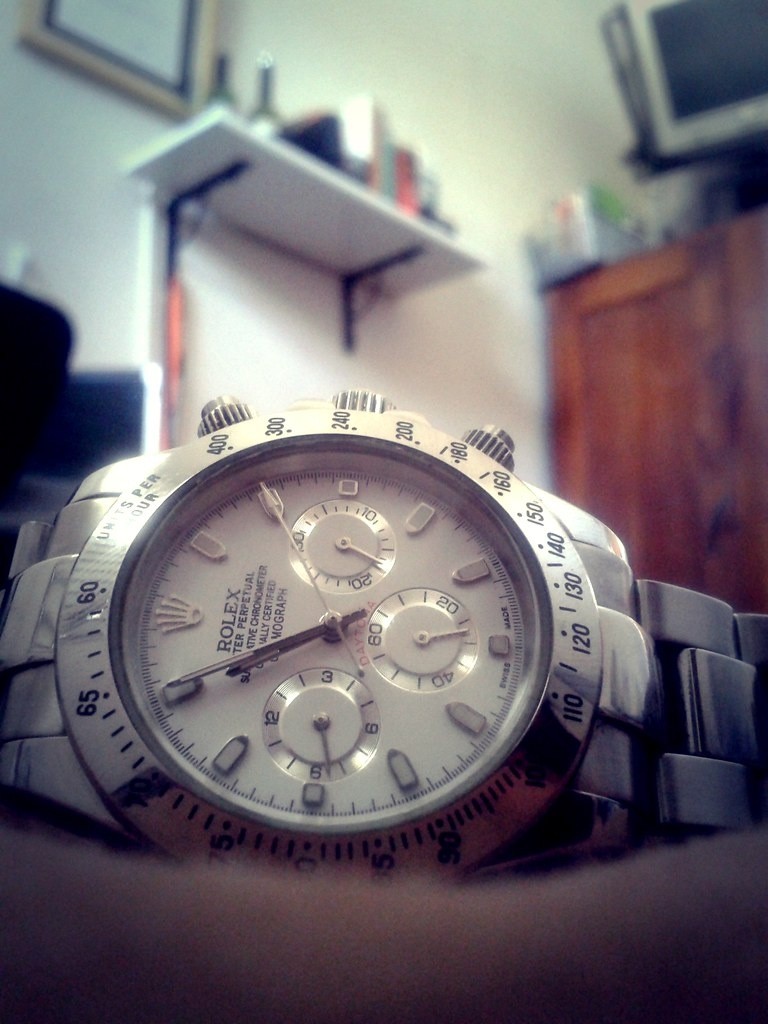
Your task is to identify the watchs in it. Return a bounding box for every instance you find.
[1,385,766,877]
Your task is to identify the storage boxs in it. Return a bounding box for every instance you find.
[528,215,644,295]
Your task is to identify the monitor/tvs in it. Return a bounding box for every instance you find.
[624,0,768,158]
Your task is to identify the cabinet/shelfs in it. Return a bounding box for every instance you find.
[540,211,768,616]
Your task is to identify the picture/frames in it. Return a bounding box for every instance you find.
[21,0,216,120]
